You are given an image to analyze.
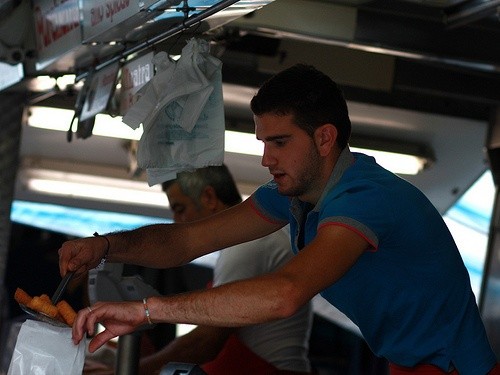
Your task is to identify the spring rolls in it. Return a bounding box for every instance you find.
[14,288,79,326]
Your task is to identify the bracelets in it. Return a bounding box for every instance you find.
[93,232,110,269]
[143,298,152,327]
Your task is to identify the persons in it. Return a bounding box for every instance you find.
[58,64,500,375]
[138,162,312,375]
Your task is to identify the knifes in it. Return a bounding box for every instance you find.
[52,270,73,305]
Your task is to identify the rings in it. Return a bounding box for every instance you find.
[87,306,91,313]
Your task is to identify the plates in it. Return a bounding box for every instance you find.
[20,303,69,329]
